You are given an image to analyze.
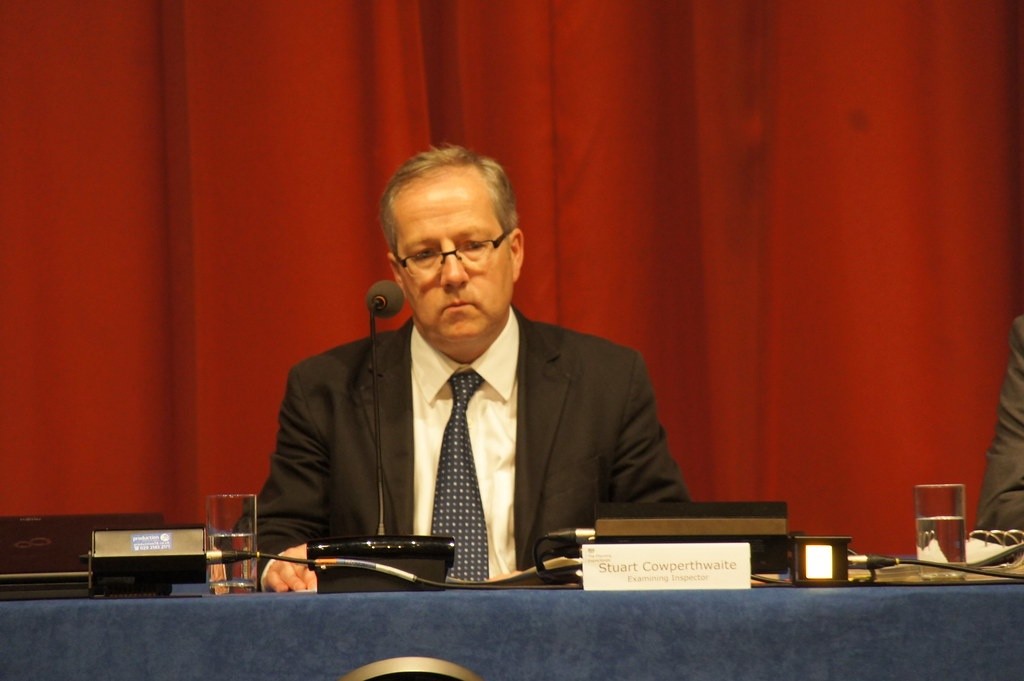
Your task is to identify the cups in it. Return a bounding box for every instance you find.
[912,483,968,565]
[203,493,258,595]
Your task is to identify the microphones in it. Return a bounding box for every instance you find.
[305,279,454,594]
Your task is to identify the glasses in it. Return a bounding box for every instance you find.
[395,228,509,281]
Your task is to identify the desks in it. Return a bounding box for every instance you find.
[0,582,1024,681]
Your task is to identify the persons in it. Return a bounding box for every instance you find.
[974,314,1024,548]
[221,142,689,592]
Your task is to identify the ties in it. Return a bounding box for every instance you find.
[430,369,491,582]
